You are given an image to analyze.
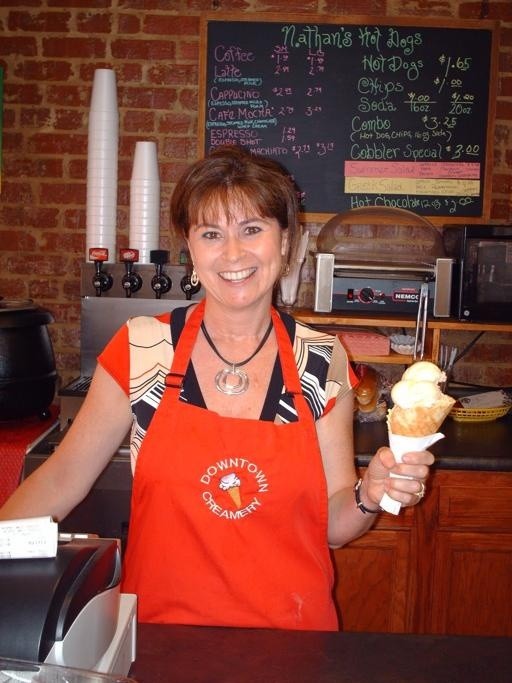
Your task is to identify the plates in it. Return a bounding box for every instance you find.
[390,334,421,355]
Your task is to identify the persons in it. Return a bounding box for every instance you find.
[1,147,436,633]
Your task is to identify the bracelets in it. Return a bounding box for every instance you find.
[352,476,386,516]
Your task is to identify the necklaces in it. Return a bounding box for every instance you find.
[198,314,274,396]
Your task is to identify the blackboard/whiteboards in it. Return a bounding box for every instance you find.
[197,12,500,226]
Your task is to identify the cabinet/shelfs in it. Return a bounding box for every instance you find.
[416,467,512,639]
[328,466,416,637]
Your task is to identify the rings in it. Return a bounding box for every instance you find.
[412,482,426,499]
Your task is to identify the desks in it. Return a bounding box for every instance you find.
[131,622,512,683]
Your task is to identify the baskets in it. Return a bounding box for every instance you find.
[448,399,512,422]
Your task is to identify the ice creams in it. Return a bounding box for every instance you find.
[387,360,459,436]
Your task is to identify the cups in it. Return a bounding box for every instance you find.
[86,68,160,263]
